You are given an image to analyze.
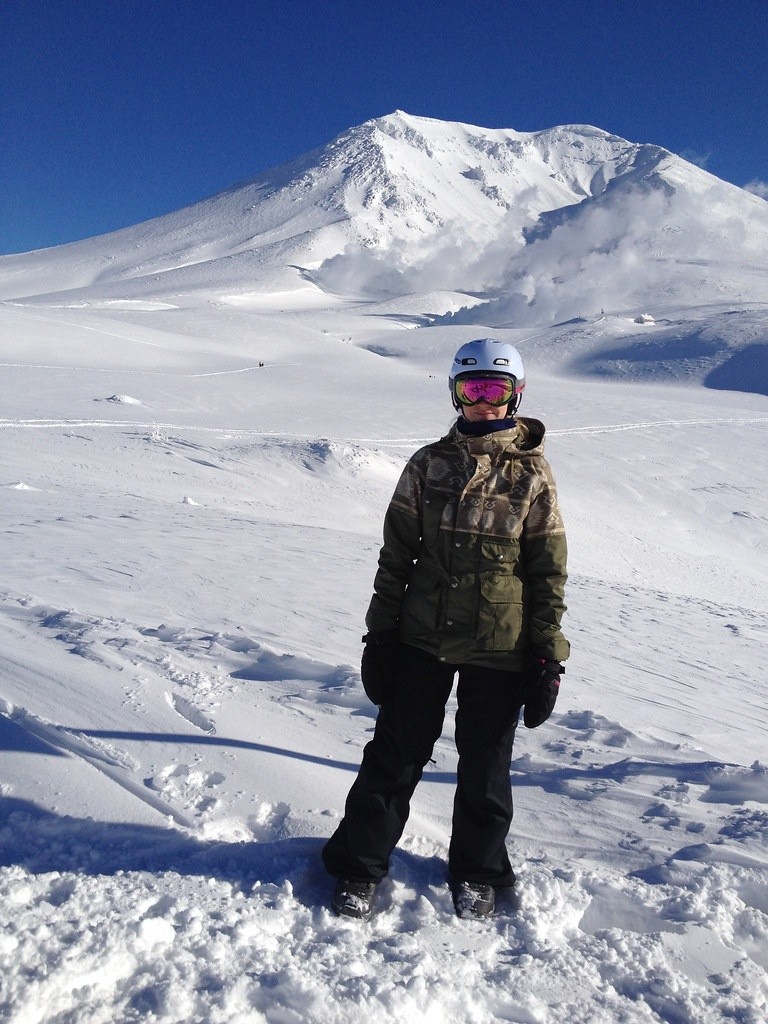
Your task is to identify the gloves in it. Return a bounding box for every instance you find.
[361,645,405,706]
[522,661,565,728]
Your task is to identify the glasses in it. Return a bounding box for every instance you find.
[449,372,526,406]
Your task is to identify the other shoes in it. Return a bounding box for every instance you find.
[332,876,376,921]
[456,879,495,919]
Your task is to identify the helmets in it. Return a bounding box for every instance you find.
[450,339,524,417]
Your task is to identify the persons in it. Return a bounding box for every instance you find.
[321,337,573,922]
